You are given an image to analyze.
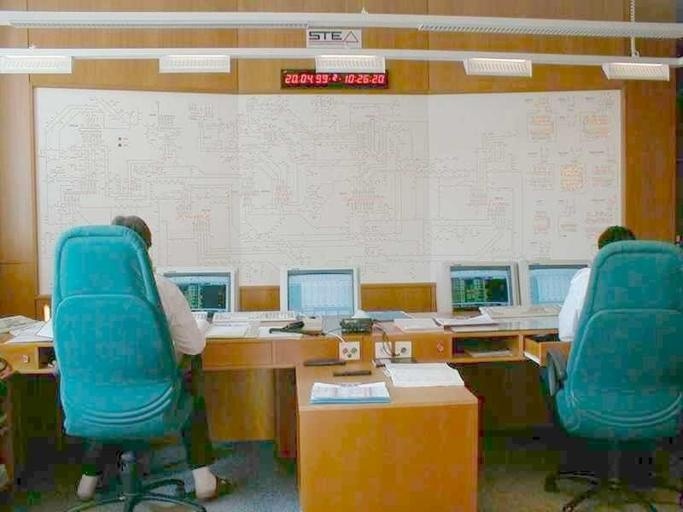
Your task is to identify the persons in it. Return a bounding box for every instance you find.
[74,212,239,503]
[554,222,665,489]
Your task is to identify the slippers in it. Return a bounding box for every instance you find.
[198,477,234,501]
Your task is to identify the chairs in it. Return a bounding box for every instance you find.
[46,221,212,511]
[542,236,680,512]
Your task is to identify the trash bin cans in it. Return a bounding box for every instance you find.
[463,383,486,465]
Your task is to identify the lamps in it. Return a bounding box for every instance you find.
[0,0,682,86]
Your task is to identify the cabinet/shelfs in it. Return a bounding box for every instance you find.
[289,358,485,512]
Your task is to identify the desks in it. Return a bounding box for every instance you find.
[0,312,365,490]
[364,301,573,371]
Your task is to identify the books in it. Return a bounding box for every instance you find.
[476,302,562,330]
[431,312,500,328]
[193,316,249,338]
[448,325,500,334]
[308,381,391,407]
[463,340,511,358]
[392,315,446,336]
[384,360,466,388]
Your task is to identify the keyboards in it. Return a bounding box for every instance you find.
[213,312,295,322]
[480,305,565,317]
[190,311,207,322]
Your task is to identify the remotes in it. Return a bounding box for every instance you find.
[306,359,346,365]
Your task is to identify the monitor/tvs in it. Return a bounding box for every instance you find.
[281,267,359,317]
[520,259,589,304]
[439,261,519,312]
[158,267,239,314]
